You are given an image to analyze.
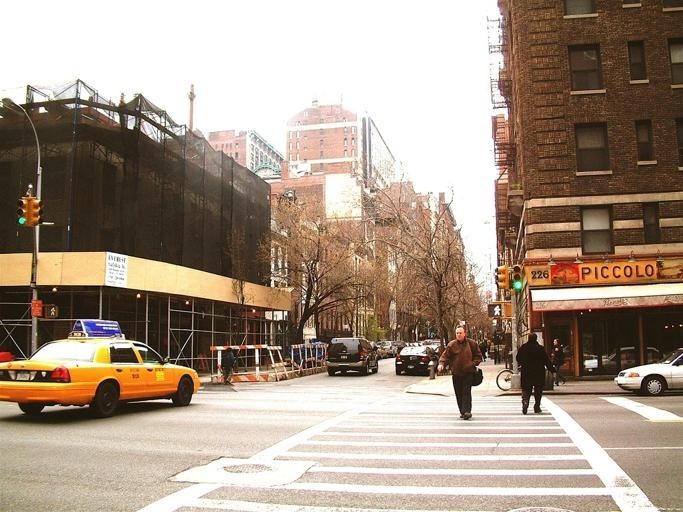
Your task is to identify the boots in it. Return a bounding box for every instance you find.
[534,407,542,413]
[555,377,567,386]
[460,413,472,419]
[522,403,528,415]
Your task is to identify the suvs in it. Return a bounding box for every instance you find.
[325,336,378,376]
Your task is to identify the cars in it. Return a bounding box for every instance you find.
[614,346,682,395]
[0,318,201,418]
[394,345,440,376]
[369,338,449,359]
[583,345,666,375]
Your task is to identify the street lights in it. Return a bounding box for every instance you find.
[2,97,43,359]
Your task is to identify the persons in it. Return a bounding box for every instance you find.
[221,346,236,384]
[437,325,482,420]
[516,333,556,415]
[551,338,567,386]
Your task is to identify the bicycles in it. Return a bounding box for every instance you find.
[496,354,513,391]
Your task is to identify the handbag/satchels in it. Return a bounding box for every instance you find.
[544,374,554,391]
[472,366,483,386]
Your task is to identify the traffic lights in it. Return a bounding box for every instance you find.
[494,264,522,293]
[486,301,512,319]
[16,196,43,230]
